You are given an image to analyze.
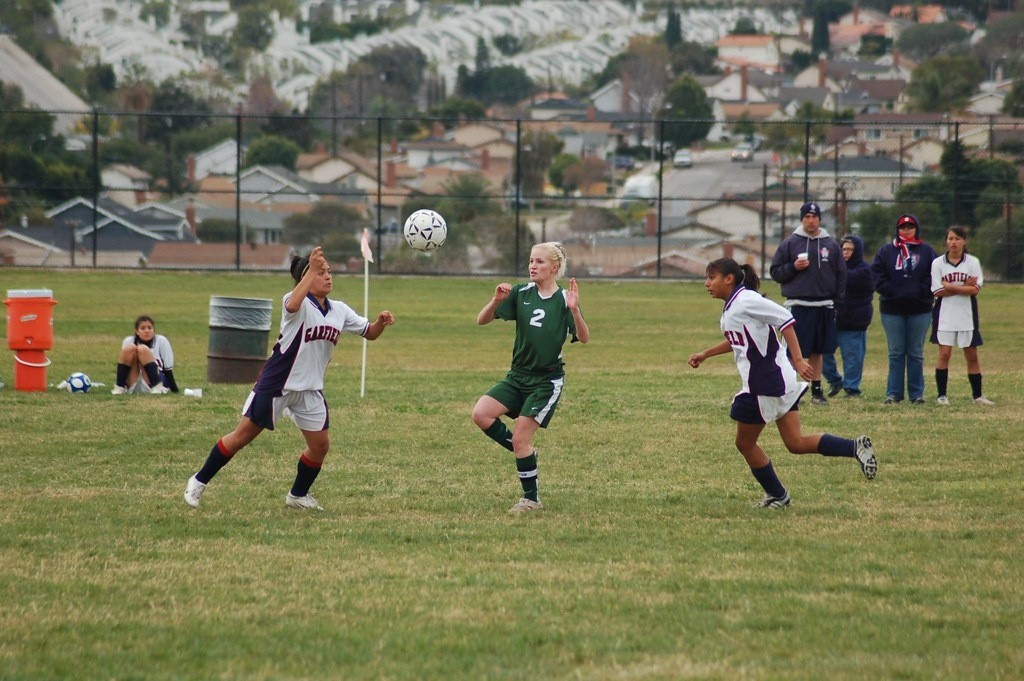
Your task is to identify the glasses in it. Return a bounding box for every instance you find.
[899,223,915,229]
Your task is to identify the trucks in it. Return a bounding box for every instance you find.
[618,174,658,210]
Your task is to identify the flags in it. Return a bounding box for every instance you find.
[359,231,374,264]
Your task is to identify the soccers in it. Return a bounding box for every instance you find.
[66,372,92,394]
[404,209,447,251]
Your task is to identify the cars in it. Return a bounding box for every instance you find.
[673,148,693,166]
[731,134,763,161]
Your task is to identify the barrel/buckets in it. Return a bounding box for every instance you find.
[3,290,58,351]
[206,295,273,385]
[12,347,48,391]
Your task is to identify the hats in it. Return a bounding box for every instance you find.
[800,203,821,220]
[898,217,916,226]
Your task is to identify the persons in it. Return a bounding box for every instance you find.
[871,214,938,404]
[110,316,180,395]
[770,202,846,404]
[821,234,873,397]
[687,258,878,511]
[472,241,589,513]
[184,246,395,510]
[930,225,993,405]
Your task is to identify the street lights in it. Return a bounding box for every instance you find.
[990,55,1008,94]
[650,103,671,176]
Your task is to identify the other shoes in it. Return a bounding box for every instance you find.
[973,396,994,406]
[827,382,843,396]
[811,397,827,407]
[884,397,899,405]
[111,385,127,395]
[845,387,859,400]
[150,384,163,394]
[912,398,925,405]
[936,395,949,405]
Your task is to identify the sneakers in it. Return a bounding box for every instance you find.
[286,490,324,510]
[854,435,877,480]
[184,473,207,506]
[509,499,542,513]
[752,490,790,509]
